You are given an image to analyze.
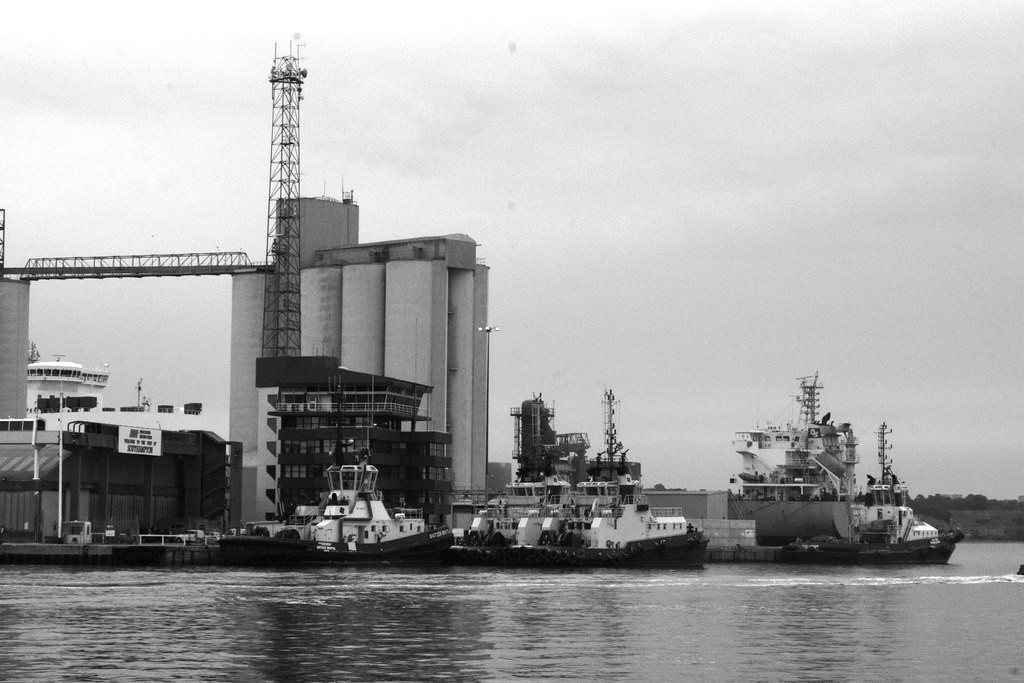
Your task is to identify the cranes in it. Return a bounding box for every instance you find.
[19,35,305,360]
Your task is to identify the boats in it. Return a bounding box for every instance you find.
[449,472,709,563]
[213,385,454,572]
[475,390,709,569]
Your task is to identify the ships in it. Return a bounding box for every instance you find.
[621,409,965,565]
